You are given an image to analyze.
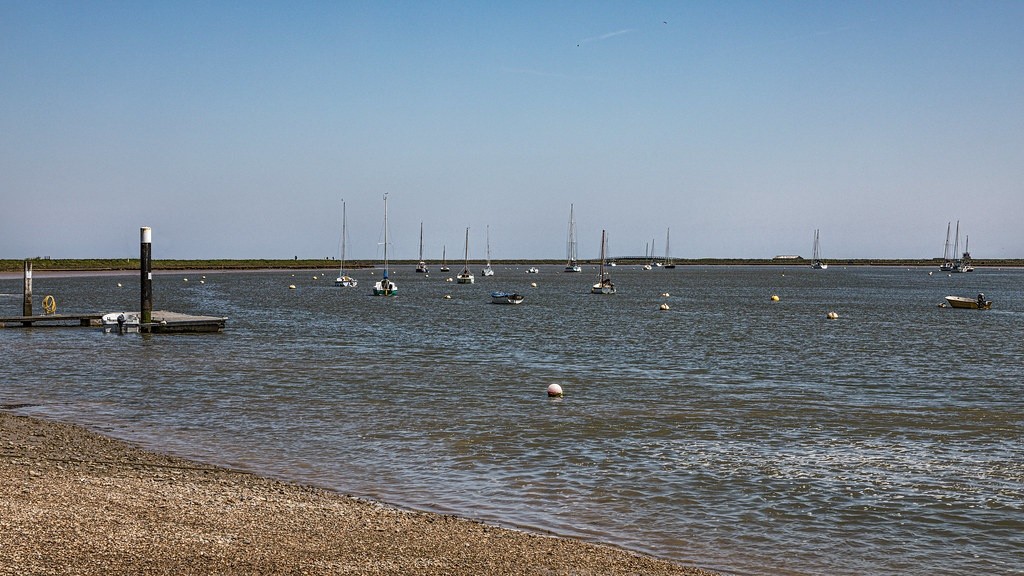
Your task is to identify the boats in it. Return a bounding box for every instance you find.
[525,267,538,273]
[945,293,992,310]
[101,313,139,335]
[491,290,524,305]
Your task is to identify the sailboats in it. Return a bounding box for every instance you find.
[650,239,662,267]
[564,203,582,272]
[664,227,676,269]
[457,227,475,284]
[939,220,974,273]
[810,229,827,270]
[480,225,495,276]
[642,242,652,270]
[415,223,430,273]
[591,230,617,295]
[335,201,359,287]
[441,245,450,272]
[373,192,398,296]
[604,233,616,267]
